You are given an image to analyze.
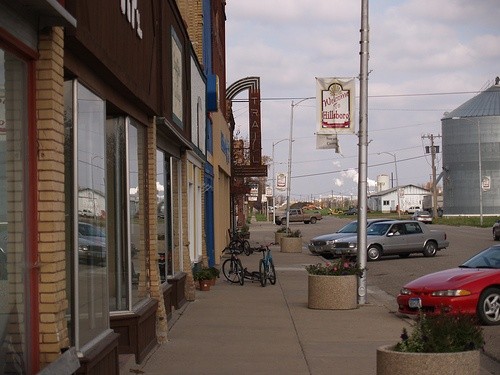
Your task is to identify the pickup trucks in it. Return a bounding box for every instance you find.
[275,208,323,225]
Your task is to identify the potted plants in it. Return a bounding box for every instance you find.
[275,228,292,244]
[240,224,250,239]
[192,263,220,291]
[280,229,302,253]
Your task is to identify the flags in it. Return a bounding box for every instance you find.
[314,75,357,136]
[275,172,288,190]
[316,133,338,149]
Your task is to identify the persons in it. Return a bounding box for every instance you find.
[390,224,401,236]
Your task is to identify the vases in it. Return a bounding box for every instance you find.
[377,346,480,375]
[308,274,358,309]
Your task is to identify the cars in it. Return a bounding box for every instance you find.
[344,207,359,216]
[396,244,500,326]
[78,221,139,266]
[410,210,433,224]
[492,219,500,241]
[405,205,420,214]
[308,219,450,262]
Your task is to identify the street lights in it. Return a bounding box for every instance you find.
[272,138,295,225]
[452,117,483,226]
[377,152,400,216]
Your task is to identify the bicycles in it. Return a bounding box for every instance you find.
[240,267,276,283]
[222,229,267,256]
[222,251,244,286]
[258,242,277,287]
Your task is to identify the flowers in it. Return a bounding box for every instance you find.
[305,259,368,278]
[392,303,486,353]
